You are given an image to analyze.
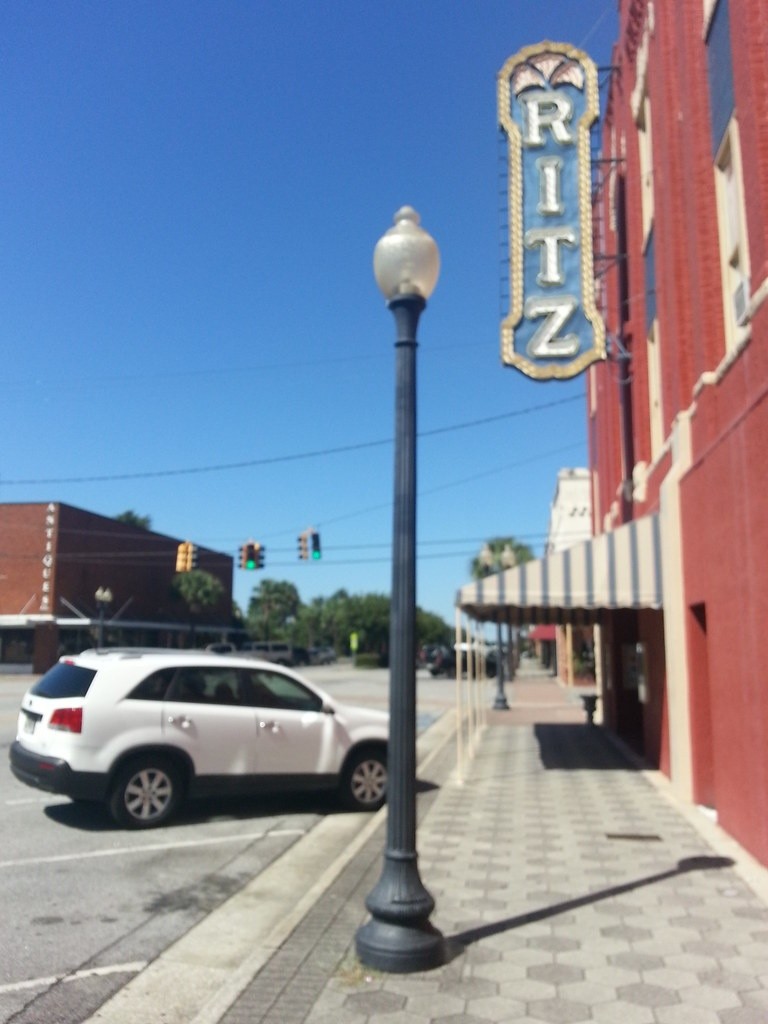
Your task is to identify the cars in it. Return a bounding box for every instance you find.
[422,638,503,677]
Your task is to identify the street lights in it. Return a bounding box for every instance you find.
[95,587,113,644]
[353,205,447,972]
[479,540,513,712]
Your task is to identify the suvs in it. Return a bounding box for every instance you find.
[9,643,391,827]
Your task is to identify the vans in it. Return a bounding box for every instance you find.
[238,642,294,665]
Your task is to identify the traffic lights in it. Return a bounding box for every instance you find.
[297,536,307,560]
[311,534,321,560]
[186,545,200,570]
[238,542,264,570]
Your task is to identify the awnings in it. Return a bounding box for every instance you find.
[453,507,664,787]
